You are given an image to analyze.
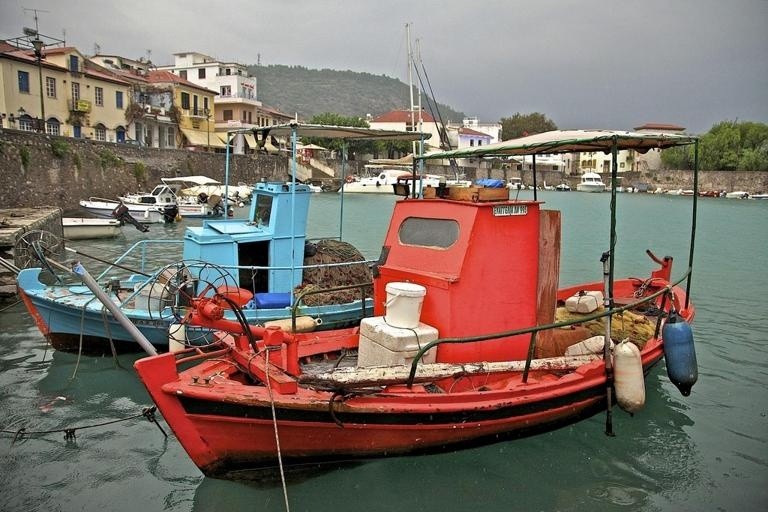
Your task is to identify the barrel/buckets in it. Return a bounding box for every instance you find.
[383,281,426,329]
[134,282,169,312]
